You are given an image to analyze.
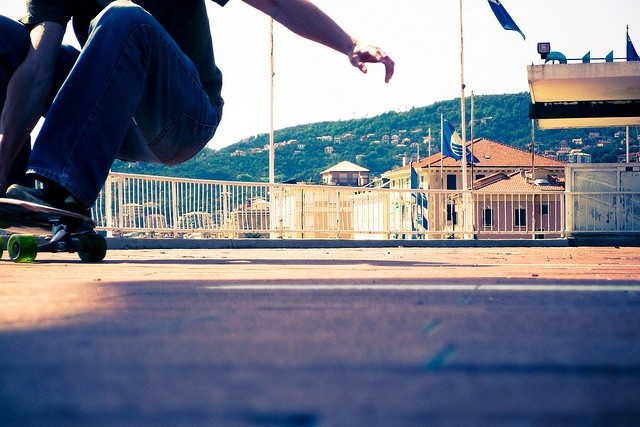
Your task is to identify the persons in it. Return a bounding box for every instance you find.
[0,1,396,220]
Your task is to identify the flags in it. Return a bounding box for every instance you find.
[409,158,428,241]
[441,115,481,165]
[626,31,640,63]
[485,0,527,42]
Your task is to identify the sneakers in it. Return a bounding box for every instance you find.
[6,181,96,218]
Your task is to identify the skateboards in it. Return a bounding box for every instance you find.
[0,197,106,262]
